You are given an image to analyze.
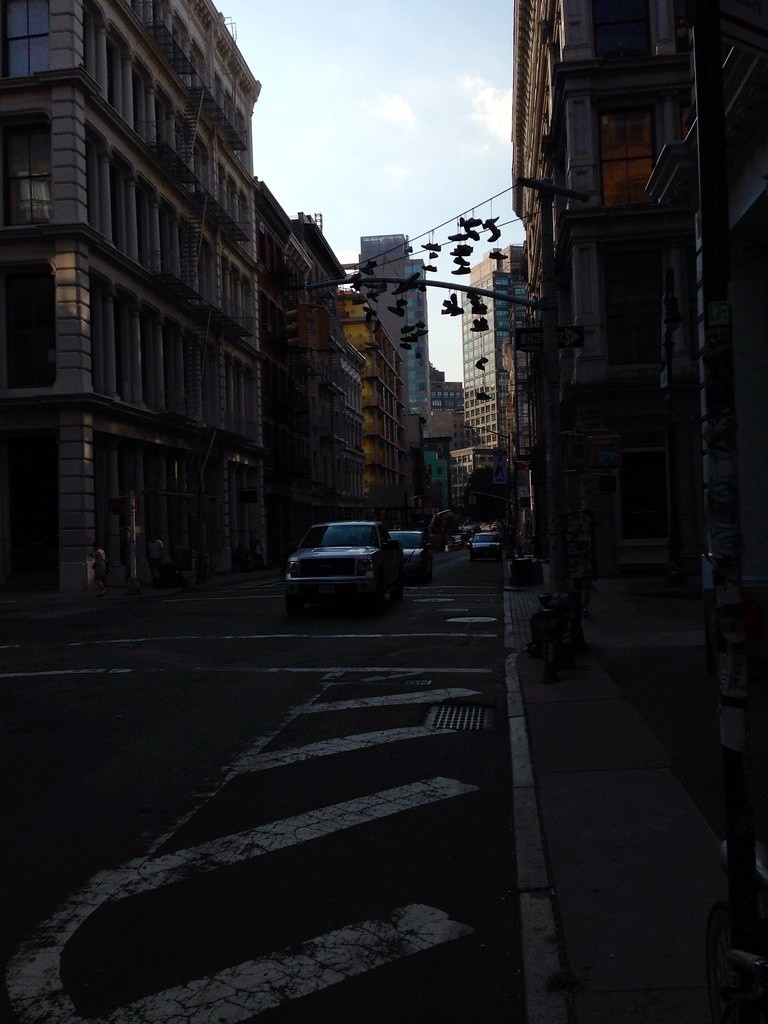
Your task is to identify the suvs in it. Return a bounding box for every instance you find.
[284,521,404,612]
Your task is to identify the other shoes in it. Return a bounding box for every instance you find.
[96,590,106,597]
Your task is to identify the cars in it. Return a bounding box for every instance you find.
[466,533,503,562]
[453,534,462,543]
[388,530,435,584]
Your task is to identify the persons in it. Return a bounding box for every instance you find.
[148,536,164,567]
[92,549,110,597]
[568,537,597,617]
[254,540,265,568]
